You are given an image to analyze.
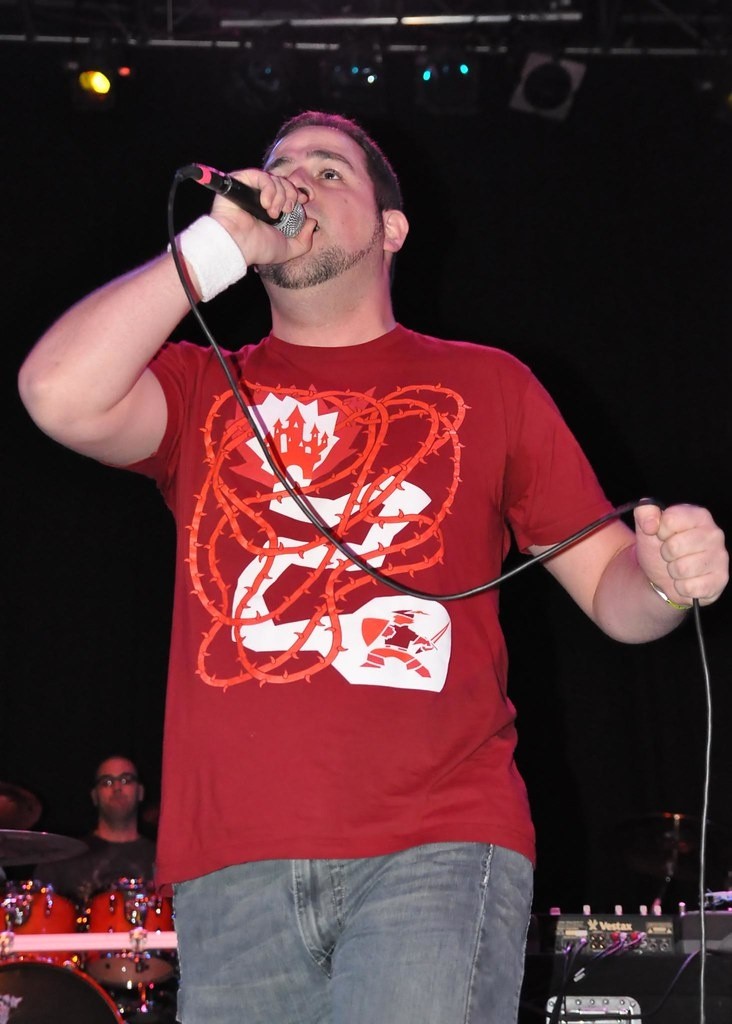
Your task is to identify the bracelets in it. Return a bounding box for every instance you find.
[649,575,693,614]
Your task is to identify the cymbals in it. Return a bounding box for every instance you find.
[0,782,42,829]
[0,828,83,869]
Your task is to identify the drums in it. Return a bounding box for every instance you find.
[0,955,125,1023]
[0,881,88,1011]
[86,881,178,988]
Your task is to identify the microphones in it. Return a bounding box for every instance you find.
[189,163,306,238]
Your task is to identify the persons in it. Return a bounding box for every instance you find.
[30,755,160,897]
[20,108,731,1024]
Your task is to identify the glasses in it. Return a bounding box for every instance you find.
[94,773,142,791]
